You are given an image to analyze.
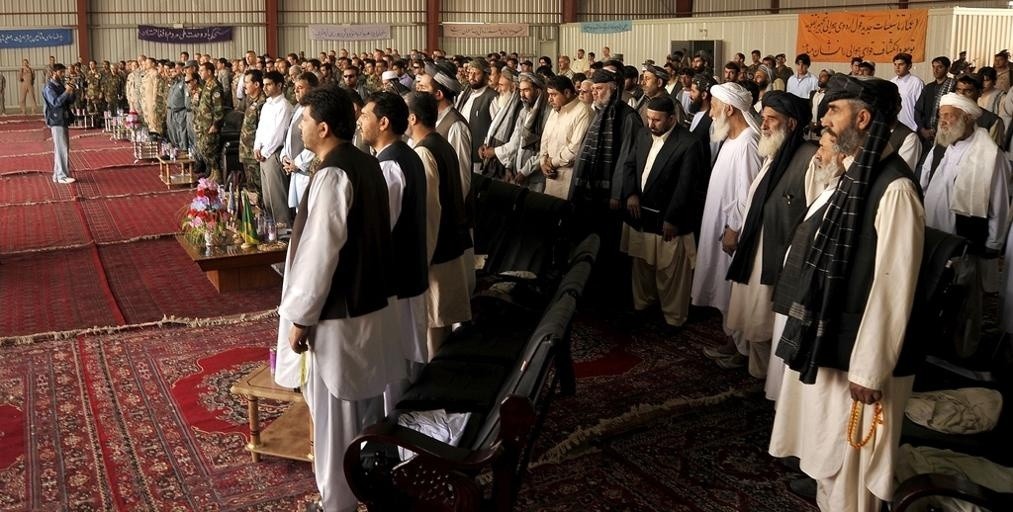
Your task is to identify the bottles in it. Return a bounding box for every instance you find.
[234,185,243,223]
[256,212,277,242]
[226,183,235,215]
[269,346,275,377]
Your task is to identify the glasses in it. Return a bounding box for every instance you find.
[413,66,420,69]
[344,75,355,78]
[185,79,193,84]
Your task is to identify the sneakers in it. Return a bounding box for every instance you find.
[702,344,735,359]
[715,352,748,369]
[307,502,323,512]
[58,177,76,184]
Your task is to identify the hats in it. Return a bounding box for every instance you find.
[182,60,198,69]
[647,97,674,112]
[382,70,400,79]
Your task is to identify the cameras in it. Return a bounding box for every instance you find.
[62,75,88,94]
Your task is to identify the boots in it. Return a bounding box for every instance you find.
[193,160,222,185]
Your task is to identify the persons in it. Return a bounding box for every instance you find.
[17,59,37,114]
[124,51,720,341]
[43,63,76,184]
[276,60,482,511]
[0,69,7,117]
[45,56,128,129]
[690,50,1012,509]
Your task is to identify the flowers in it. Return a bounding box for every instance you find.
[180,174,232,244]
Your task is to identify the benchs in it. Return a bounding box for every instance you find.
[891,339,1012,510]
[341,231,604,510]
[918,228,968,339]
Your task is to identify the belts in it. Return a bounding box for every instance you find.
[187,108,193,112]
[173,107,186,113]
